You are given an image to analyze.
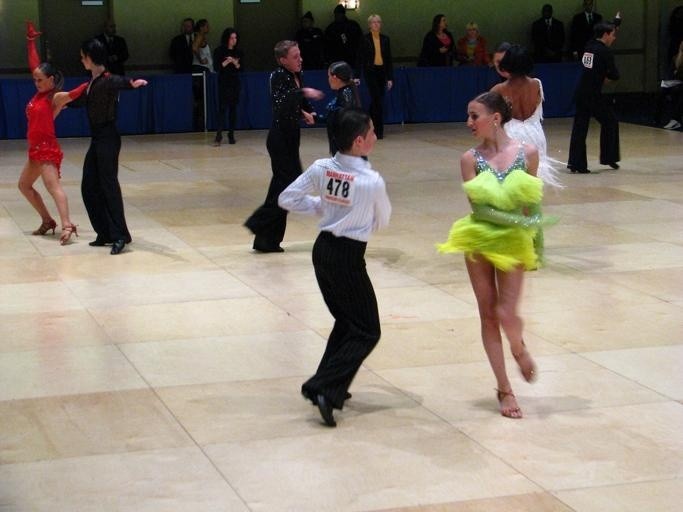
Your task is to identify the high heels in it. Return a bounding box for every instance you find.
[227,132,235,144]
[60,223,80,245]
[32,220,57,236]
[216,133,223,146]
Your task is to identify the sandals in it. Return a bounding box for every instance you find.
[510,340,537,384]
[495,385,523,419]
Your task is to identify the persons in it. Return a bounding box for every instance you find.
[566,12,623,173]
[293,12,326,68]
[302,61,365,161]
[569,1,604,62]
[658,42,683,130]
[241,40,323,253]
[434,91,541,421]
[490,46,573,198]
[323,5,361,68]
[191,19,213,75]
[455,22,492,68]
[169,18,194,75]
[512,70,515,71]
[16,16,90,246]
[58,37,148,254]
[472,43,544,104]
[276,109,393,426]
[212,27,243,144]
[415,14,455,67]
[531,4,564,62]
[93,21,128,75]
[358,14,391,139]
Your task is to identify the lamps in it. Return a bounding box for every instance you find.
[338,0,360,9]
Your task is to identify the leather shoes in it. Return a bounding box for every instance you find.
[110,232,133,253]
[600,159,620,169]
[88,234,116,245]
[300,377,336,427]
[264,247,284,253]
[569,165,590,174]
